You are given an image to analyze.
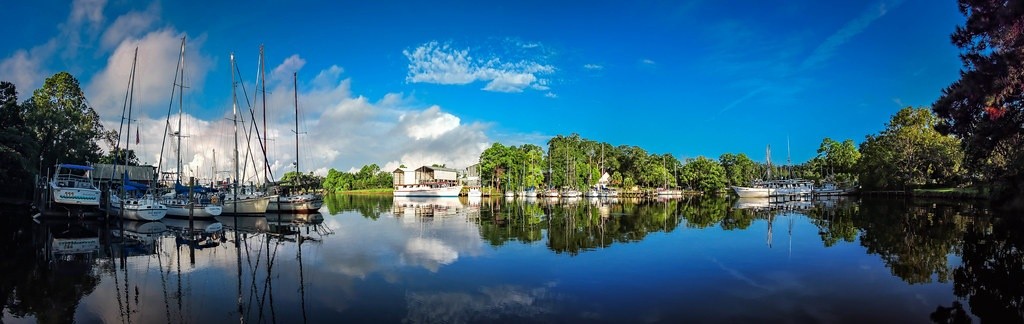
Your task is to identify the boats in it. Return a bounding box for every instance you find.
[49,163,102,206]
[40,210,100,259]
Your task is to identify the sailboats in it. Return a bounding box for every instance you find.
[213,213,307,324]
[103,212,168,324]
[143,33,223,217]
[162,216,225,321]
[265,209,335,324]
[99,46,168,222]
[232,43,324,215]
[198,52,272,216]
[473,143,843,208]
